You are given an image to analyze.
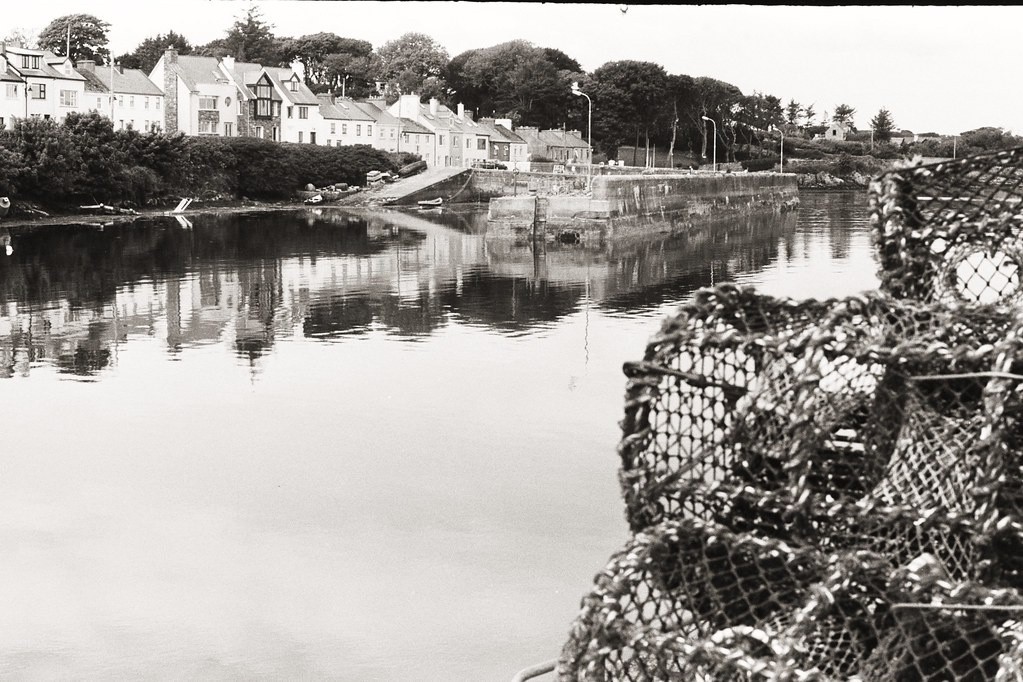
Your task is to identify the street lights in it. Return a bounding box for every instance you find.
[572,89,592,192]
[773,128,783,172]
[871,124,874,150]
[701,116,716,172]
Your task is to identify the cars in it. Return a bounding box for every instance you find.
[471,158,481,168]
[481,158,507,170]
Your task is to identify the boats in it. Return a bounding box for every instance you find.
[418,197,443,206]
[296,183,360,201]
